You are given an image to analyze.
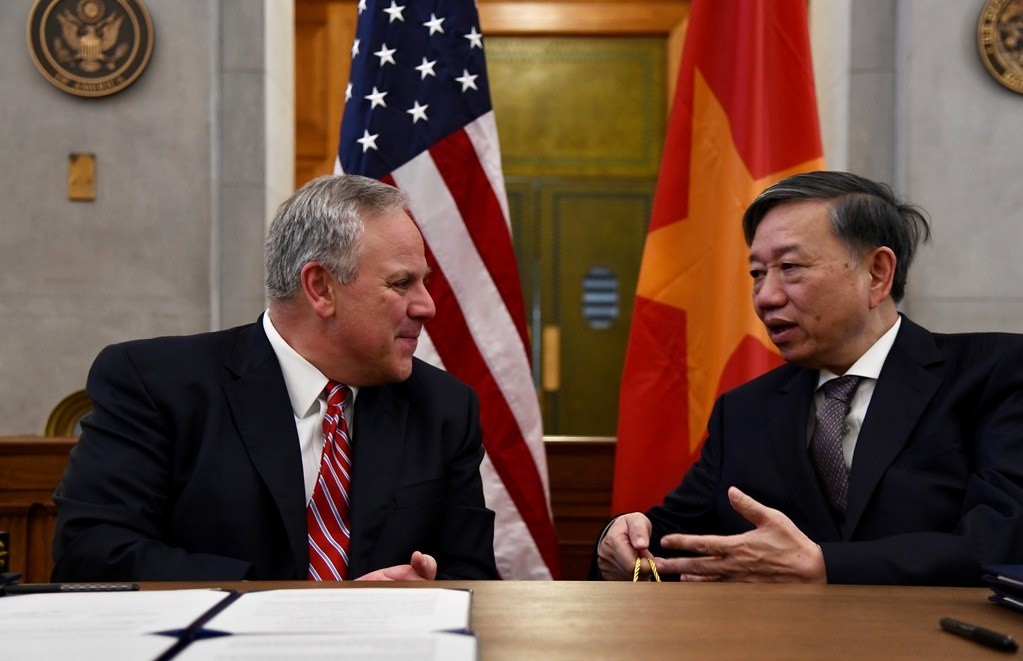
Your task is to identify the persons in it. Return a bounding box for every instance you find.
[51,174,503,583]
[594,171,1023,587]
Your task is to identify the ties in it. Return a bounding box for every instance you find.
[809,374,861,514]
[308,378,354,582]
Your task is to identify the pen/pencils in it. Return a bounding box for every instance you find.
[939,616,1019,653]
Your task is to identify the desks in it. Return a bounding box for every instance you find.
[18,582,1023,661]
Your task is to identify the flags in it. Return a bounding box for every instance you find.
[332,0,563,582]
[610,0,827,517]
